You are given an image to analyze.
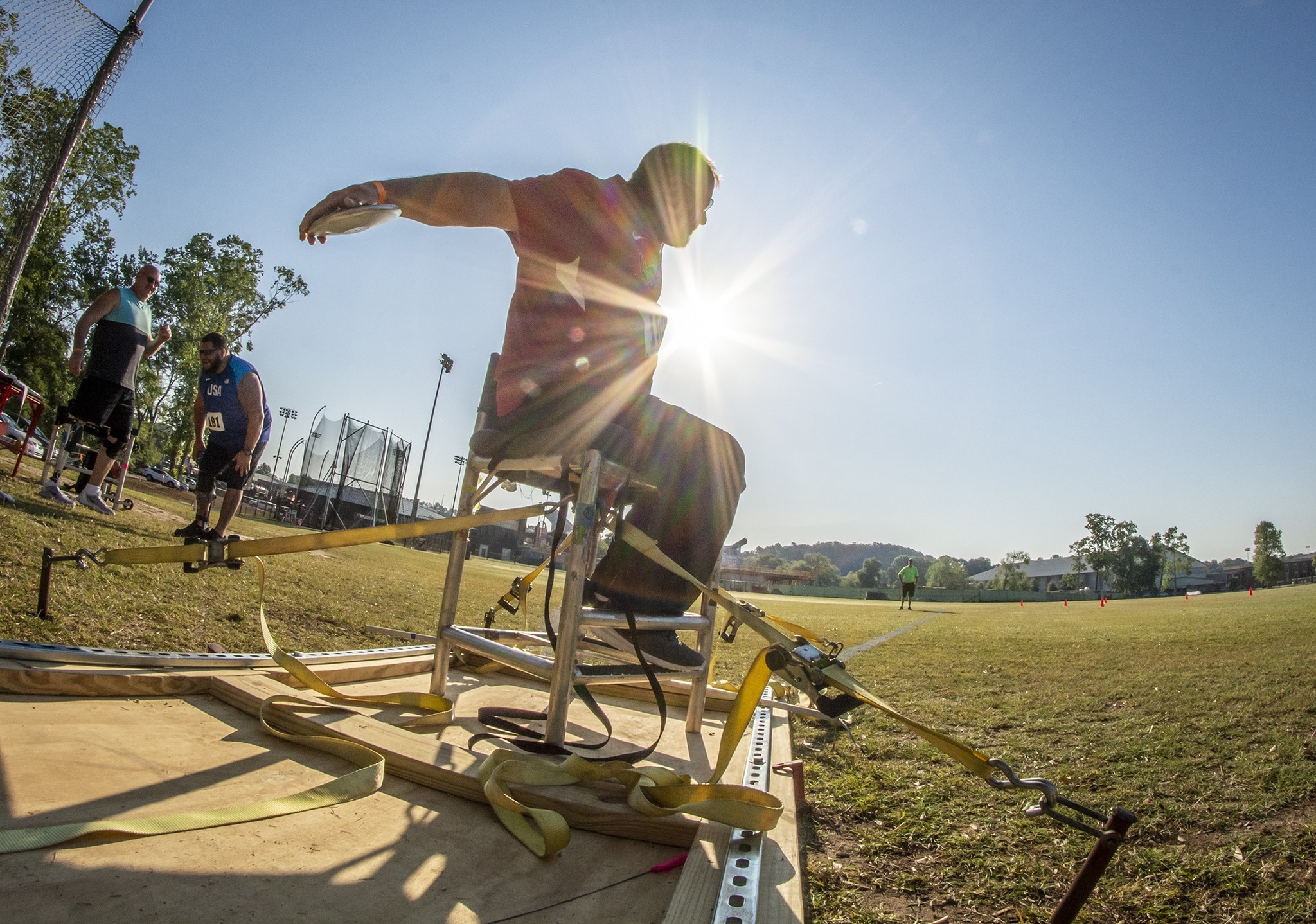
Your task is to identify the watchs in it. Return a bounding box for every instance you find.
[241,446,254,456]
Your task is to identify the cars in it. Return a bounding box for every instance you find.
[0,412,44,460]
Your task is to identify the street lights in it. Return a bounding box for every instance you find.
[405,353,455,549]
[1244,547,1251,566]
[1305,546,1311,556]
[267,407,298,500]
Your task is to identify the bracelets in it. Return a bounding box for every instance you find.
[73,348,82,353]
[370,179,387,206]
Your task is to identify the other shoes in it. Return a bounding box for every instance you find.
[559,586,706,670]
[76,488,115,515]
[175,521,209,538]
[908,607,912,610]
[193,529,226,539]
[38,480,76,507]
[898,606,903,609]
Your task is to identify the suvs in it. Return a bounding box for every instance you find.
[137,464,198,492]
[5,411,58,462]
[248,499,277,514]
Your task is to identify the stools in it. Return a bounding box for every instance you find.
[40,403,140,512]
[0,365,46,479]
[429,352,721,757]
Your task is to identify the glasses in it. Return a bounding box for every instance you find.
[142,271,160,287]
[681,178,714,212]
[199,349,217,355]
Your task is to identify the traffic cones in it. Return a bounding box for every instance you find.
[1105,597,1107,603]
[1249,587,1253,595]
[1185,592,1189,600]
[1020,599,1024,606]
[1099,597,1104,607]
[1064,597,1067,606]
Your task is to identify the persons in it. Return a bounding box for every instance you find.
[63,449,123,496]
[300,140,746,671]
[174,332,272,542]
[898,559,919,610]
[41,268,172,517]
